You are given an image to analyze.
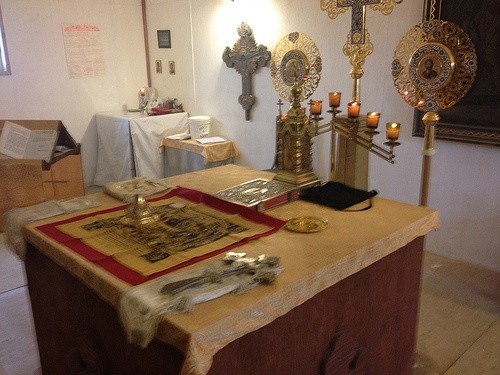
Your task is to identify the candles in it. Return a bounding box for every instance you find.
[367,112,379,127]
[386,122,399,139]
[330,92,340,106]
[349,102,360,118]
[312,101,321,114]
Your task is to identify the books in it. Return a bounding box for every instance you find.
[0,121,57,162]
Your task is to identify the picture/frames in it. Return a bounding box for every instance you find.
[157,29,171,48]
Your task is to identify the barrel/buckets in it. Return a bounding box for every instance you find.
[188,115,211,140]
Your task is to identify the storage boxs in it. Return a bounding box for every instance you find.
[0,115,86,236]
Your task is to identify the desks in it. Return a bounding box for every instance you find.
[93,111,189,188]
[160,138,239,178]
[6,164,440,375]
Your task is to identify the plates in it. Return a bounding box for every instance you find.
[284,215,329,233]
[108,178,164,196]
